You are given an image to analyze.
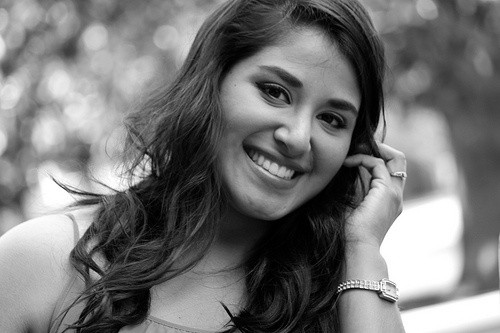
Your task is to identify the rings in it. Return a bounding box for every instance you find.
[390,171,408,179]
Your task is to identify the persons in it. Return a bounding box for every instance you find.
[1,0,408,333]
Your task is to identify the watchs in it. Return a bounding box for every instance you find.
[336,279,400,303]
